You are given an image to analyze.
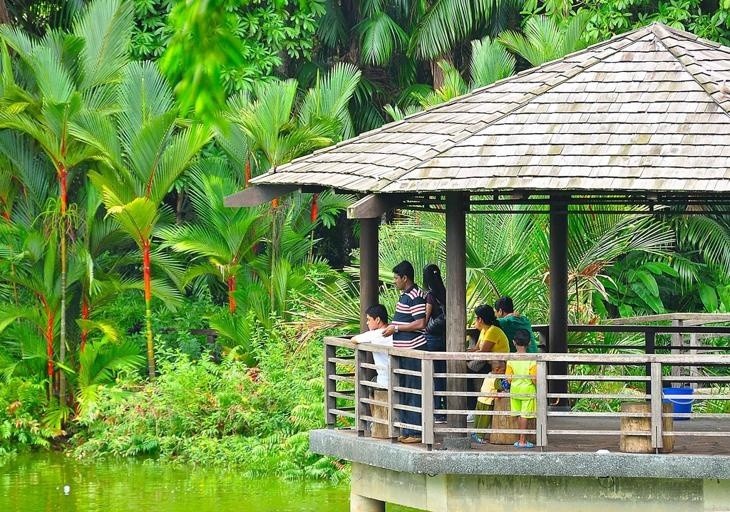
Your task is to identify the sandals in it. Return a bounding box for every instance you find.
[514,440,534,448]
[471,432,487,444]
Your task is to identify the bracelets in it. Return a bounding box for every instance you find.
[395,325,399,333]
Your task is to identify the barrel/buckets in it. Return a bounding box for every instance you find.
[663,388,694,421]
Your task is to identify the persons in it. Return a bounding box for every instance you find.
[382,260,429,443]
[351,304,394,436]
[421,263,447,424]
[466,304,511,424]
[472,360,508,444]
[493,296,540,354]
[505,329,539,448]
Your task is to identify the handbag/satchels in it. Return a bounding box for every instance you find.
[426,291,446,335]
[466,360,486,372]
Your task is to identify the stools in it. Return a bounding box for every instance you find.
[472,379,484,391]
[620,403,673,453]
[490,397,536,444]
[371,390,388,439]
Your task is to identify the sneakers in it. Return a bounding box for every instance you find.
[398,435,422,443]
[434,415,447,424]
[467,415,474,422]
